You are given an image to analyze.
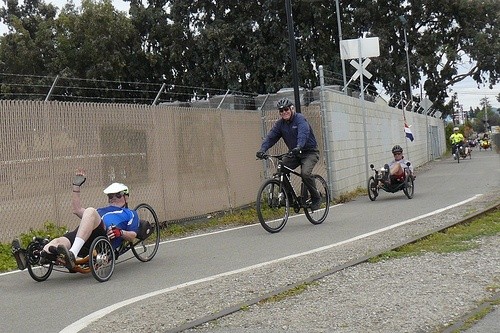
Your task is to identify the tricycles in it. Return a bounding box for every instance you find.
[452,140,472,164]
[367,164,414,201]
[479,141,492,151]
[11,203,160,282]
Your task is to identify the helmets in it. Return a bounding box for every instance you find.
[104,182,129,195]
[454,127,459,131]
[392,145,403,152]
[277,99,293,108]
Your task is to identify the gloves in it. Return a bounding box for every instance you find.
[257,152,264,158]
[292,148,300,155]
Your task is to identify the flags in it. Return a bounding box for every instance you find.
[404,119,415,142]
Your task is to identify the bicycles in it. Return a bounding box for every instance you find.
[256,151,330,234]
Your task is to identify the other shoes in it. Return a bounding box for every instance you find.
[13,238,27,270]
[280,197,293,206]
[56,244,75,268]
[311,196,323,210]
[384,164,389,179]
[453,154,455,157]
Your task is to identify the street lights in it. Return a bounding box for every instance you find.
[399,14,415,112]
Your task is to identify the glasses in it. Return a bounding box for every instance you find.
[108,193,121,199]
[395,152,402,155]
[279,108,289,112]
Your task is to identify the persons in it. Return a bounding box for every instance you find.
[377,144,413,189]
[256,98,321,212]
[449,128,464,160]
[13,168,140,271]
[462,132,491,156]
